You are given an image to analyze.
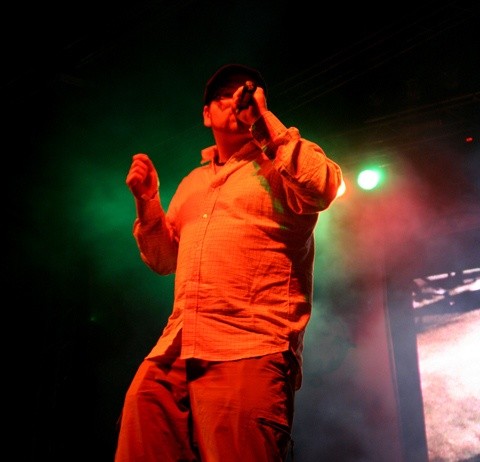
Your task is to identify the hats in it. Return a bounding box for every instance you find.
[203,62,267,108]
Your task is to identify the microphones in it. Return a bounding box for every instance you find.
[237,78,258,111]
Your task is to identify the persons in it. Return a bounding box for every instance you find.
[107,60,342,461]
